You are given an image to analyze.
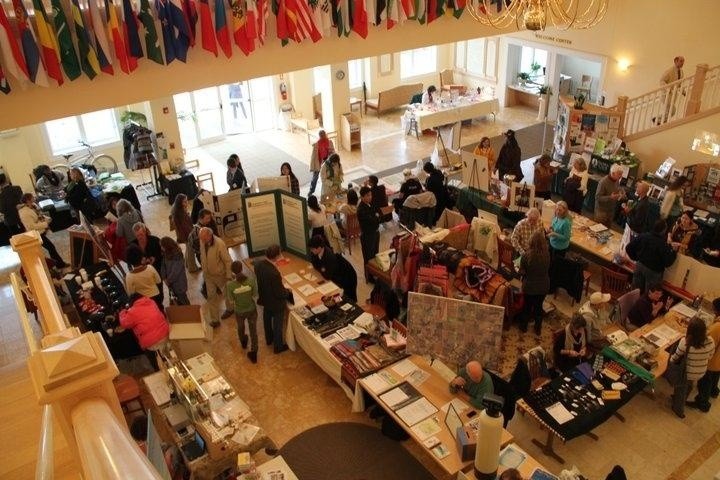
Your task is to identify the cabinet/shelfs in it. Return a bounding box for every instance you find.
[340,113,362,154]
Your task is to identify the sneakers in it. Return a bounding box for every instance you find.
[267,340,273,344]
[247,351,257,364]
[221,309,234,319]
[274,345,288,352]
[209,320,220,328]
[241,334,248,348]
[694,396,710,412]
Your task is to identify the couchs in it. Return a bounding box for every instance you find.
[365,84,423,120]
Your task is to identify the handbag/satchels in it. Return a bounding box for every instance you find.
[667,354,687,385]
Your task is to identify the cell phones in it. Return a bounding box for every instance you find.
[178,428,187,434]
[467,411,476,418]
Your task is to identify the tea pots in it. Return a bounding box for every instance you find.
[692,294,702,309]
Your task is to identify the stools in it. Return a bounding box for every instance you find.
[111,373,148,418]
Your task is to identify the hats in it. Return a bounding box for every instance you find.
[590,291,611,304]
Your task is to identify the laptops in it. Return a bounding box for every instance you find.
[181,431,206,461]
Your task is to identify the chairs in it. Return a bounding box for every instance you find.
[439,66,467,95]
[575,72,594,101]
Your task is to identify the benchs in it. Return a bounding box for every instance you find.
[273,420,437,479]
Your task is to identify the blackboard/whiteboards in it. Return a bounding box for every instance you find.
[406,292,505,372]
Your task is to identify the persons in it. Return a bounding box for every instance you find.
[650,55,687,126]
[422,85,436,106]
[306,130,718,416]
[227,82,247,119]
[1,154,300,373]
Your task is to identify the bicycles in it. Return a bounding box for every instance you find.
[49,141,118,186]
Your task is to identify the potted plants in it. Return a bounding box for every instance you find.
[530,60,542,77]
[539,86,550,99]
[516,71,531,88]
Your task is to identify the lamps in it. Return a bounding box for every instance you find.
[465,0,612,35]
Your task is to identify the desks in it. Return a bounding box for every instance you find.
[157,168,199,206]
[240,177,720,480]
[509,70,572,111]
[35,180,142,234]
[406,93,502,141]
[137,353,277,480]
[61,260,144,360]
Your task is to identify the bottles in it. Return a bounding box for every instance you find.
[74,268,122,323]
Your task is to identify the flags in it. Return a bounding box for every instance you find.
[0,0,527,93]
